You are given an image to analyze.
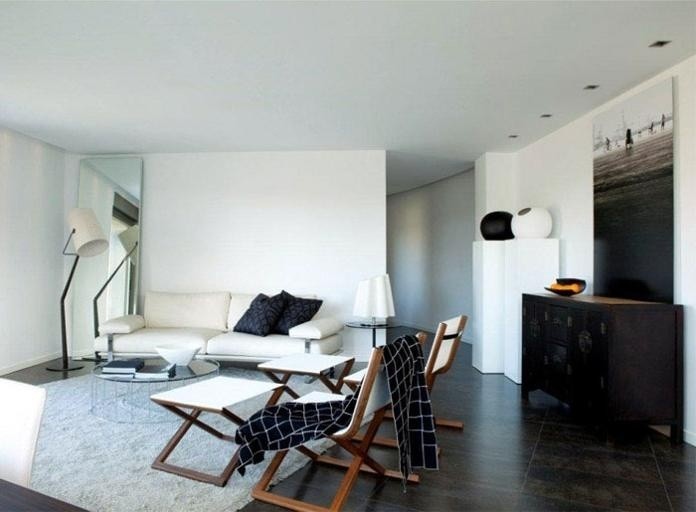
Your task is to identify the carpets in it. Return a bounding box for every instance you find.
[7,366,376,512]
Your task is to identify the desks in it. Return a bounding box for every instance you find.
[147,377,287,491]
[253,352,356,407]
[344,320,400,348]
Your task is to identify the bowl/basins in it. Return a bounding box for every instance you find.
[153,346,201,367]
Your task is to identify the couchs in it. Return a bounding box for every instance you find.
[95,290,351,384]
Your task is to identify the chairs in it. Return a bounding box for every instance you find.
[248,331,431,512]
[338,313,468,456]
[0,374,49,487]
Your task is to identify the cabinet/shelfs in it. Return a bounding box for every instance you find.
[504,236,559,386]
[518,289,685,455]
[472,239,506,375]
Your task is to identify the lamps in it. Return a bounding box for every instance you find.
[79,222,140,361]
[43,204,108,372]
[353,273,396,327]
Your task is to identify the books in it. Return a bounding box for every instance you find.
[100,360,176,380]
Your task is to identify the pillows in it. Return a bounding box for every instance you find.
[233,293,284,335]
[276,289,325,335]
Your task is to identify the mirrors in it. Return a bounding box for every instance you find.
[69,154,140,360]
